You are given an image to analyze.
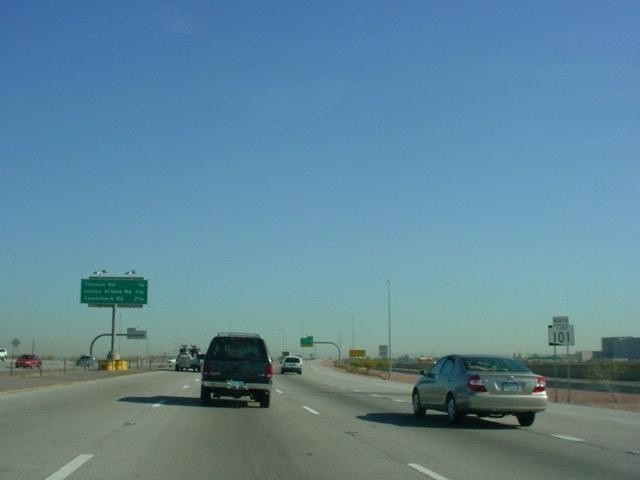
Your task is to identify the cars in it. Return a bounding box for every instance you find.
[410,350,550,429]
[76,355,97,368]
[0,347,9,360]
[15,353,43,369]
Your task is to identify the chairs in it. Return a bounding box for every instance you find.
[215,350,230,360]
[241,352,257,361]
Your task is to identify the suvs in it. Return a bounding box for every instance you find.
[168,328,304,408]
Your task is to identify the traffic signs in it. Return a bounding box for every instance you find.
[300,336,314,348]
[80,278,149,306]
